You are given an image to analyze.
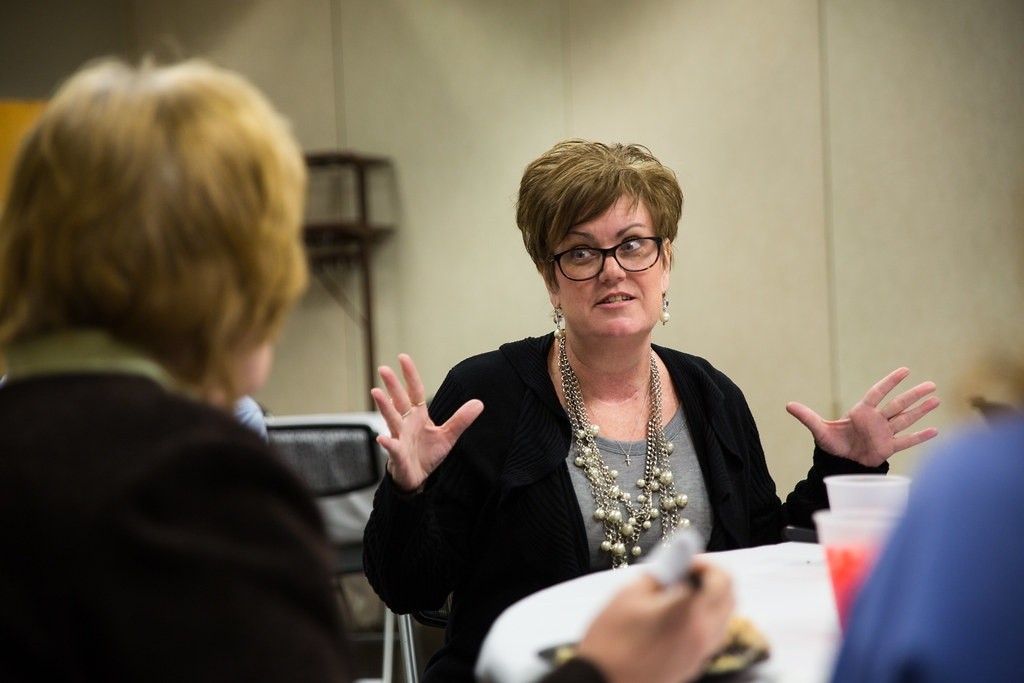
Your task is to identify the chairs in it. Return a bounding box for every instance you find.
[260,412,419,683]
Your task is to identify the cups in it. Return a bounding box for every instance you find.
[812,470,914,639]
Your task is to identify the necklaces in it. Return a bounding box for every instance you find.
[557,329,690,570]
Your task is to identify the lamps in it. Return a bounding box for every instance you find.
[296,148,400,246]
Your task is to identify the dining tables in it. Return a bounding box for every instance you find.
[472,542,844,683]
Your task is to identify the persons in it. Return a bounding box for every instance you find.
[1,50,742,683]
[359,138,942,683]
[831,420,1024,683]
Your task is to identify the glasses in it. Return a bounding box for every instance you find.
[549,236,662,282]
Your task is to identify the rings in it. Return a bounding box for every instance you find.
[414,400,428,407]
[401,407,412,419]
